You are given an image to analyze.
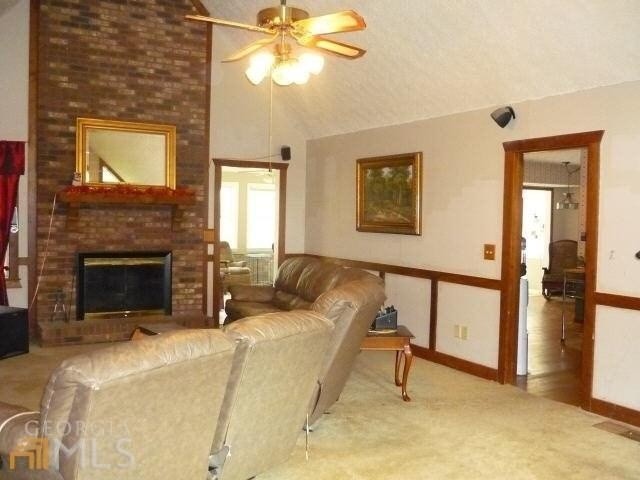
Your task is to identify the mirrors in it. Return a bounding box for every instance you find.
[75,117,177,192]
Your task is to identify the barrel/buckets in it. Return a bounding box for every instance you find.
[521,237,526,277]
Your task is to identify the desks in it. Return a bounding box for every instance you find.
[356,318,428,405]
[560,269,586,346]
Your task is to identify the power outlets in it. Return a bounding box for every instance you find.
[452,323,469,342]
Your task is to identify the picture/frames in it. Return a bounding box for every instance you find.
[354,151,423,237]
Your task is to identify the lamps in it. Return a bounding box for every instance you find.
[185,0,366,62]
[246,1,325,89]
[492,105,517,129]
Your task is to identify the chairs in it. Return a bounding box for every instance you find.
[219,241,252,296]
[541,239,586,299]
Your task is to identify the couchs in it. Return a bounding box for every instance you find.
[0,254,387,480]
